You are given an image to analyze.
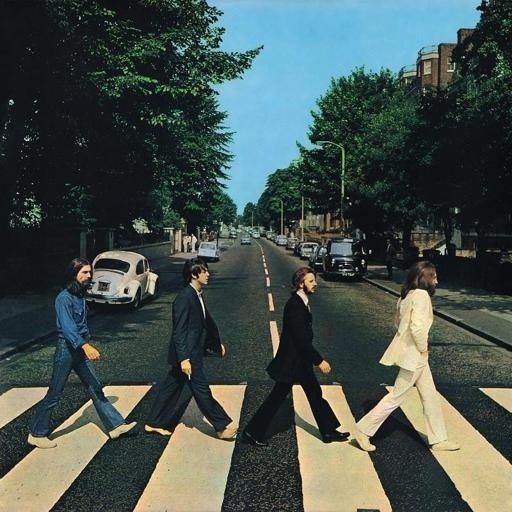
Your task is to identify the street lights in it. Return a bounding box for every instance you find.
[288,186,305,240]
[248,209,254,227]
[316,140,346,239]
[273,197,284,234]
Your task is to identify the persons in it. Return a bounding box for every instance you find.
[27,257,138,448]
[352,261,460,451]
[145,259,240,439]
[384,239,396,281]
[241,268,350,446]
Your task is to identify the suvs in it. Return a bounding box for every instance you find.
[323,236,372,279]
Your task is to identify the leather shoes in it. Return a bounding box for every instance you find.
[27,433,57,448]
[145,425,172,436]
[432,442,460,451]
[217,428,240,439]
[109,422,137,438]
[243,429,268,447]
[323,430,350,443]
[354,430,376,452]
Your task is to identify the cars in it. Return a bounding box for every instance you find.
[197,241,220,261]
[227,225,328,275]
[83,249,160,312]
